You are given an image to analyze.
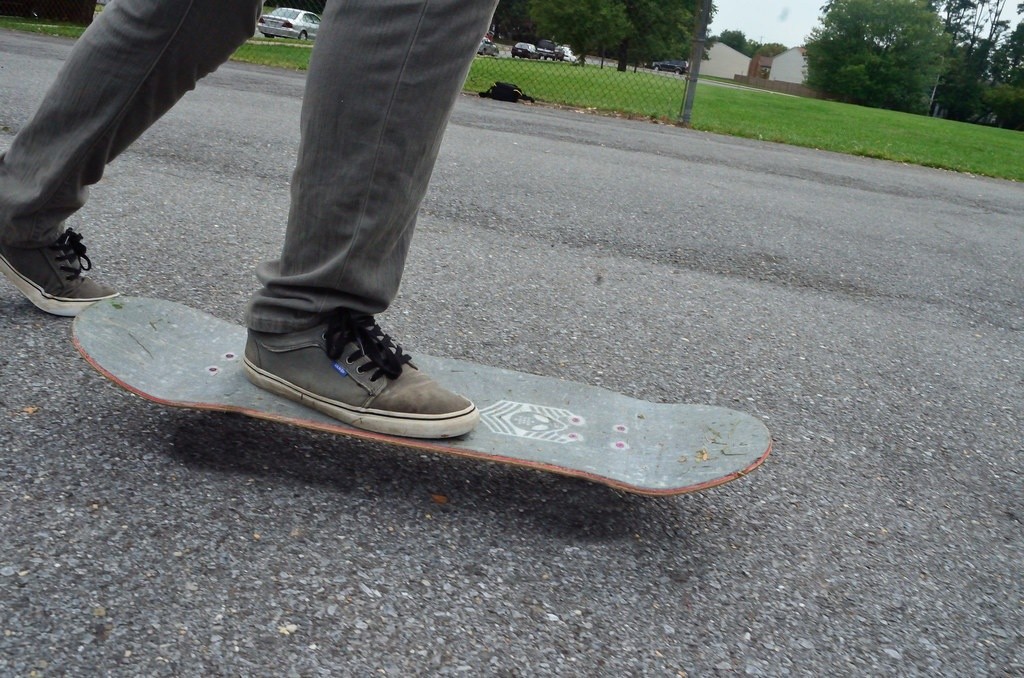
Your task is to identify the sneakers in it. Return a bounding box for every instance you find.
[244,316,479,437]
[0,227,120,316]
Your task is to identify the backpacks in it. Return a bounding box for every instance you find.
[479,82,534,104]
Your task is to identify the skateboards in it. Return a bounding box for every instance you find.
[70,294,775,498]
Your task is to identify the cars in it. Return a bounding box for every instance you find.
[651,58,689,75]
[477,37,500,58]
[563,46,578,63]
[537,39,566,62]
[511,42,541,60]
[257,7,321,41]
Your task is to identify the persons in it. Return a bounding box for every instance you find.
[0,1,497,439]
